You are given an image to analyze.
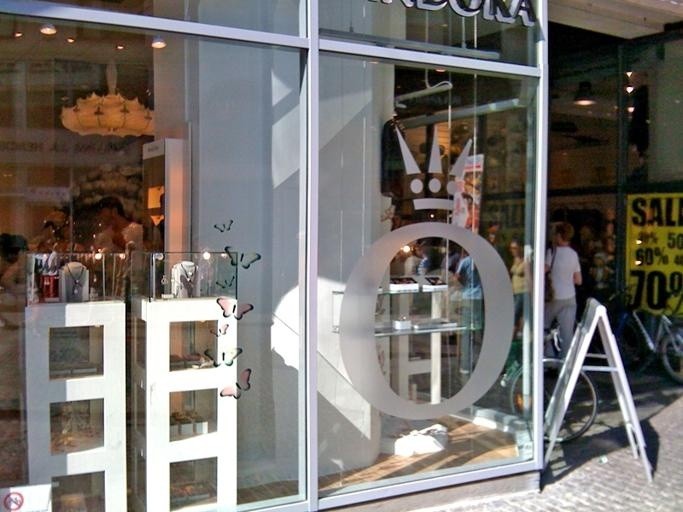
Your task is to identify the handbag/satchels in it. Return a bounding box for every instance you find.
[545,245,557,302]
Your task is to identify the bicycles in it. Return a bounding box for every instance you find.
[606,280,682,386]
[498,320,599,448]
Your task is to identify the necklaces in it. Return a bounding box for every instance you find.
[180,263,196,285]
[65,263,84,296]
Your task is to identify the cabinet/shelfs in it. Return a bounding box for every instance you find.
[383,281,446,454]
[20,251,131,512]
[131,249,239,511]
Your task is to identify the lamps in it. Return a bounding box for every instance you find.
[61,62,154,137]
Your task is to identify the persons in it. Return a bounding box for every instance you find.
[405,222,526,388]
[95,195,143,305]
[544,221,583,374]
